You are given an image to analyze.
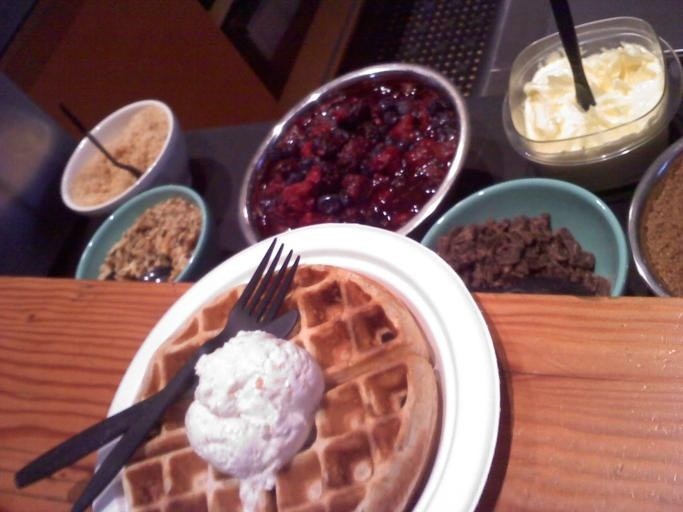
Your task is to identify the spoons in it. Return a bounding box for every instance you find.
[55,99,143,182]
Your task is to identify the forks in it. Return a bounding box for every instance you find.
[70,240,297,510]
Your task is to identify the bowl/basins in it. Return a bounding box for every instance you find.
[630,141,683,299]
[76,182,212,282]
[417,180,628,294]
[502,17,683,193]
[240,61,471,250]
[58,97,193,230]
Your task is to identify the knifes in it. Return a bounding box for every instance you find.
[13,309,296,487]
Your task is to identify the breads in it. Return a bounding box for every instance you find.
[121,263,442,512]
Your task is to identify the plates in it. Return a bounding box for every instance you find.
[87,221,503,508]
